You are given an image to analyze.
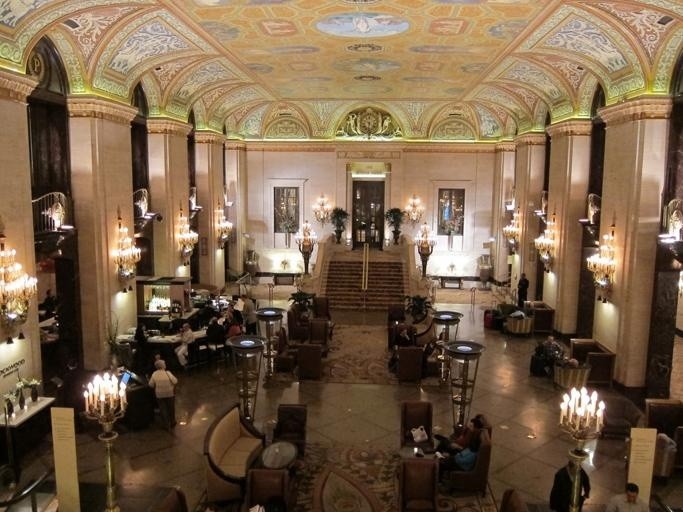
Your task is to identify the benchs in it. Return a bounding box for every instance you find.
[202,403,265,505]
[570,339,616,391]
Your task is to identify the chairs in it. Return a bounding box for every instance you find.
[440,422,492,498]
[400,455,439,511]
[242,468,289,512]
[52,294,258,430]
[596,392,682,512]
[554,361,591,393]
[272,404,307,459]
[400,401,432,447]
[272,293,451,389]
[483,300,554,336]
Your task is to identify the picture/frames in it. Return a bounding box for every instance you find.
[438,188,465,236]
[273,187,299,234]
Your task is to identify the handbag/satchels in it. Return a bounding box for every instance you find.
[174,385,176,394]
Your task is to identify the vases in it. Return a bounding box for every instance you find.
[245,250,260,285]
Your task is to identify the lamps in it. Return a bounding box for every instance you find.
[295,193,436,279]
[501,188,683,304]
[81,369,131,512]
[558,387,606,512]
[2,181,236,341]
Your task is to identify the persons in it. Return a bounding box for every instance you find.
[439,432,481,471]
[517,273,530,307]
[205,294,257,344]
[434,428,468,453]
[549,460,591,512]
[147,360,178,430]
[605,482,648,512]
[44,288,57,318]
[174,323,193,365]
[396,324,411,347]
[543,335,562,356]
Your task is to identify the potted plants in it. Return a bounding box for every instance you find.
[2,377,42,417]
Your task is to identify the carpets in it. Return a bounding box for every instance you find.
[193,443,499,511]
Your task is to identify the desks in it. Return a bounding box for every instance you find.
[440,276,461,289]
[0,395,56,487]
